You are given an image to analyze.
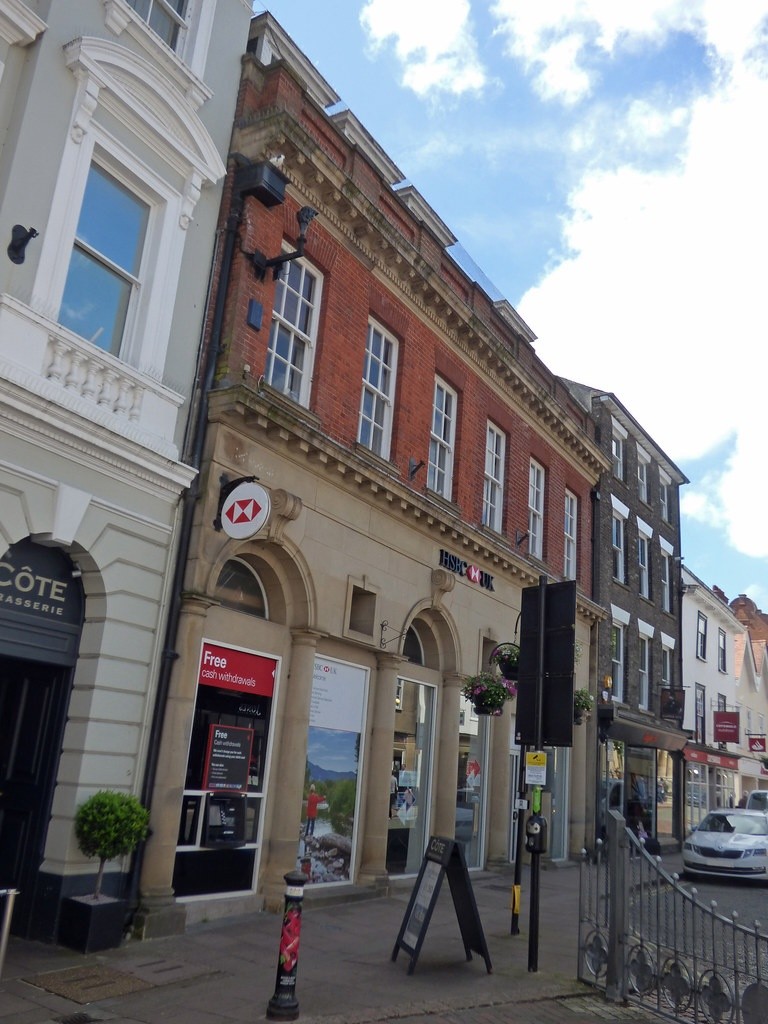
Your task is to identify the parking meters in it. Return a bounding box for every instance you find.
[524,813,546,972]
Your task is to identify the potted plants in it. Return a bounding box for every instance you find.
[56,789,151,955]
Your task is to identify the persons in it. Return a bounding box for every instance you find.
[305,784,326,836]
[738,791,749,808]
[388,770,399,820]
[658,778,664,804]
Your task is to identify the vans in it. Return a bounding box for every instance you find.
[745,790,768,812]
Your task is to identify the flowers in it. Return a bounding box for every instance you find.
[574,688,596,712]
[488,641,520,667]
[459,672,518,707]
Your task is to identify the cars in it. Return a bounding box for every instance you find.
[682,808,768,889]
[454,787,478,839]
